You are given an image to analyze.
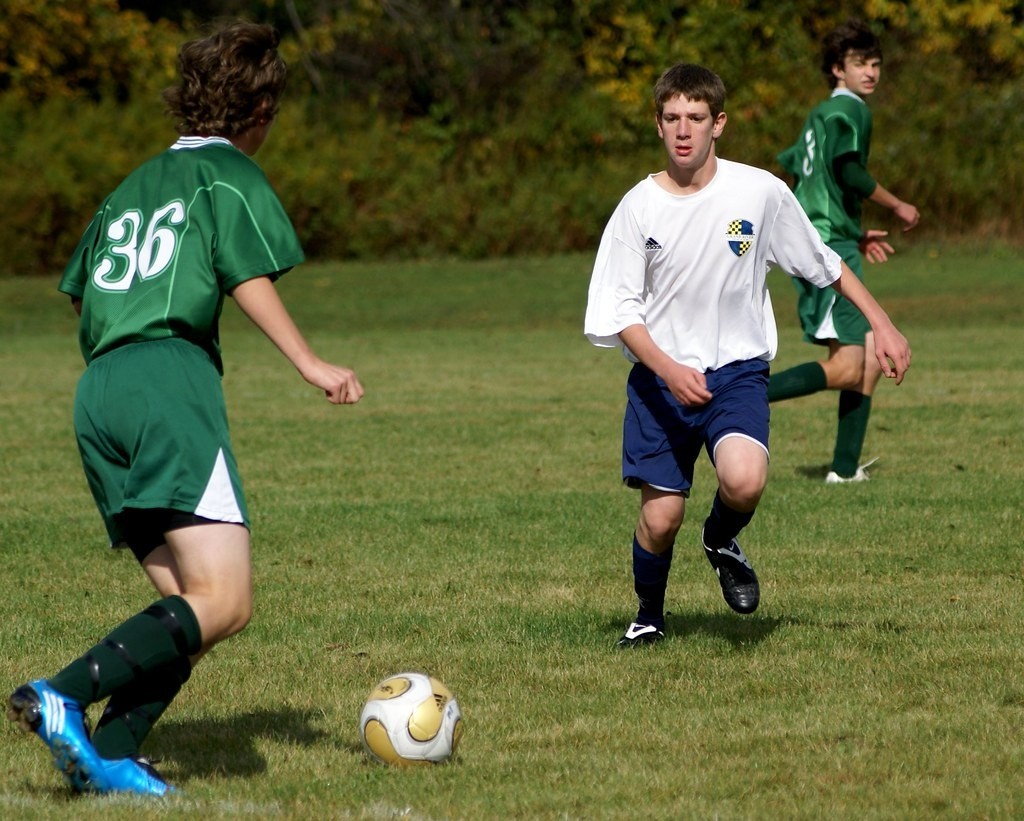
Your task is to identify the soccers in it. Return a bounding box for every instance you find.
[360,673,465,767]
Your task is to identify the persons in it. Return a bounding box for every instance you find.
[583,65,913,644]
[766,22,919,484]
[10,19,365,801]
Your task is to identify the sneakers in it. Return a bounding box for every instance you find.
[6,679,113,796]
[702,516,760,614]
[100,756,177,801]
[616,622,664,649]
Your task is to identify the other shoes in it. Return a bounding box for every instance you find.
[826,456,879,483]
